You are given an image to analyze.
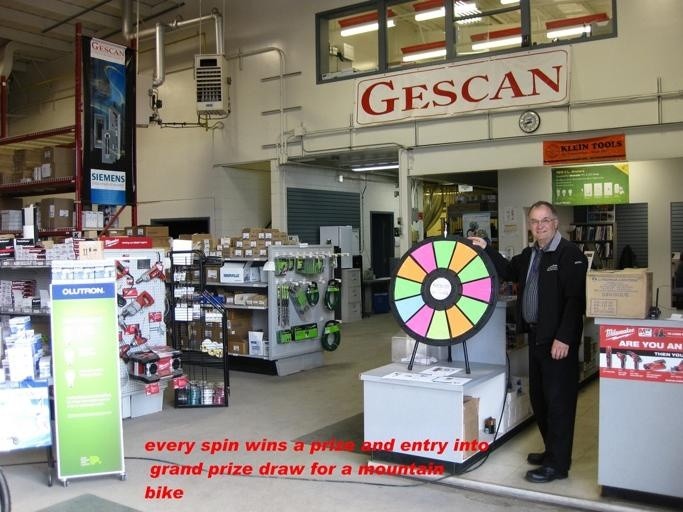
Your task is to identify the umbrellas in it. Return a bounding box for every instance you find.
[527,453,546,465]
[527,464,568,483]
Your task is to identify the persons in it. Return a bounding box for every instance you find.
[469,201,590,484]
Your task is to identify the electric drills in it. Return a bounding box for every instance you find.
[115,260,167,359]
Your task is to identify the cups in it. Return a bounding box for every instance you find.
[529,218,557,224]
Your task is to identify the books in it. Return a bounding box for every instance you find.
[42,145,76,180]
[125,226,168,247]
[42,198,73,231]
[585,267,653,318]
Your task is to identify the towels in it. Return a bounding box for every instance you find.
[373,293,389,314]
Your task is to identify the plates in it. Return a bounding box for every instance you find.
[594,307,683,500]
[359,295,599,472]
[0,122,79,237]
[0,247,166,420]
[166,244,343,376]
